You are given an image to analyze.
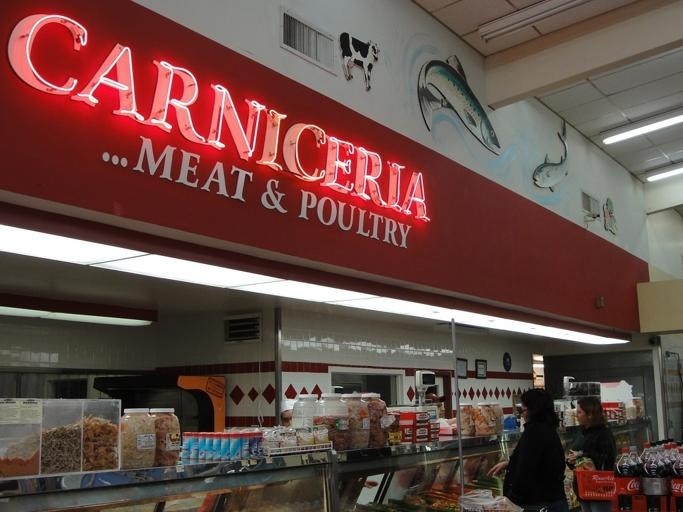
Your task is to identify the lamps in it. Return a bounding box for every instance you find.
[88,254,285,288]
[327,299,629,344]
[45,302,156,326]
[0,224,149,265]
[0,295,53,320]
[476,0,598,43]
[596,106,683,146]
[228,280,379,304]
[635,161,683,184]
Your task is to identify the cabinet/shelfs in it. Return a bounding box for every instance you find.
[498,417,654,464]
[94,374,225,433]
[335,433,503,512]
[0,450,328,512]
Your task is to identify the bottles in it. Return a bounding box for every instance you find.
[474,402,498,436]
[629,445,644,470]
[641,447,669,512]
[291,393,319,430]
[181,431,264,463]
[631,397,642,418]
[670,447,683,512]
[150,407,182,467]
[490,403,504,434]
[120,407,157,470]
[669,443,679,465]
[616,447,640,511]
[312,392,350,452]
[340,393,372,450]
[660,444,673,468]
[362,393,389,449]
[644,441,662,463]
[455,403,475,436]
[640,443,650,462]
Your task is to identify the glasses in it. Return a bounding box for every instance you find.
[517,406,527,414]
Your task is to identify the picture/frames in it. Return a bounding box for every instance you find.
[456,357,468,377]
[474,359,487,379]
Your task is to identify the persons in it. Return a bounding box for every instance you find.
[487,388,569,512]
[565,397,616,512]
